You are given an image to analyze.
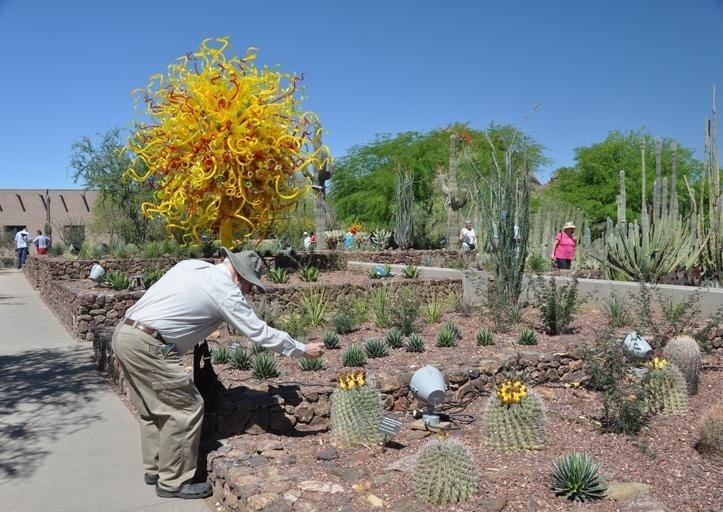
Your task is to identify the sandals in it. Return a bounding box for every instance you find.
[145,474,212,499]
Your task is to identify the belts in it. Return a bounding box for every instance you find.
[121,316,168,345]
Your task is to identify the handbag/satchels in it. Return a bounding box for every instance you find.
[194,367,219,412]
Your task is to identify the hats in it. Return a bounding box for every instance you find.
[220,246,268,295]
[563,222,576,229]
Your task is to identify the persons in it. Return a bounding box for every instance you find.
[368,232,375,244]
[458,220,479,251]
[303,230,317,251]
[111,247,325,501]
[14,227,31,269]
[550,221,576,270]
[34,229,50,256]
[341,232,354,248]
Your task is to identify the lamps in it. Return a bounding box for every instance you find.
[89,264,106,288]
[621,331,654,375]
[409,365,447,425]
